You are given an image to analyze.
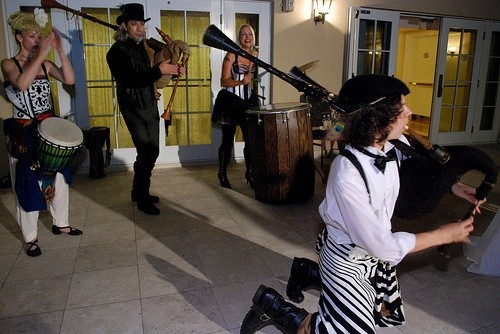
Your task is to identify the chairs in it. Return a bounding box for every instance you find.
[301,92,344,170]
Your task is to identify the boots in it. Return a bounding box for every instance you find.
[217,147,233,190]
[240,284,310,334]
[243,147,261,188]
[286,256,321,304]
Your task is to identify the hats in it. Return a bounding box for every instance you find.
[336,75,412,114]
[116,3,151,25]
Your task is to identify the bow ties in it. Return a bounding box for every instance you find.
[374,149,398,172]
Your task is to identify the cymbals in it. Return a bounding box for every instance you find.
[300,59,320,72]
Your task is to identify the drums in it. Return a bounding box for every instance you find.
[84,126,110,180]
[34,116,85,202]
[245,101,315,205]
[299,92,338,130]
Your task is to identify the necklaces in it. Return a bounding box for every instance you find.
[19,52,33,60]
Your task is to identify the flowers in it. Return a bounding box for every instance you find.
[33,7,49,27]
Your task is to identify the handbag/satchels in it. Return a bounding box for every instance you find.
[211,89,248,125]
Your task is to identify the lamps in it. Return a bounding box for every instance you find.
[312,0,333,27]
[449,45,467,61]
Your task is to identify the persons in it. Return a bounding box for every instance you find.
[106,4,185,215]
[211,24,259,189]
[1,11,83,257]
[240,74,487,334]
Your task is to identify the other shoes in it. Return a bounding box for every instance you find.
[52,225,83,235]
[137,202,160,216]
[27,239,42,257]
[133,195,160,203]
